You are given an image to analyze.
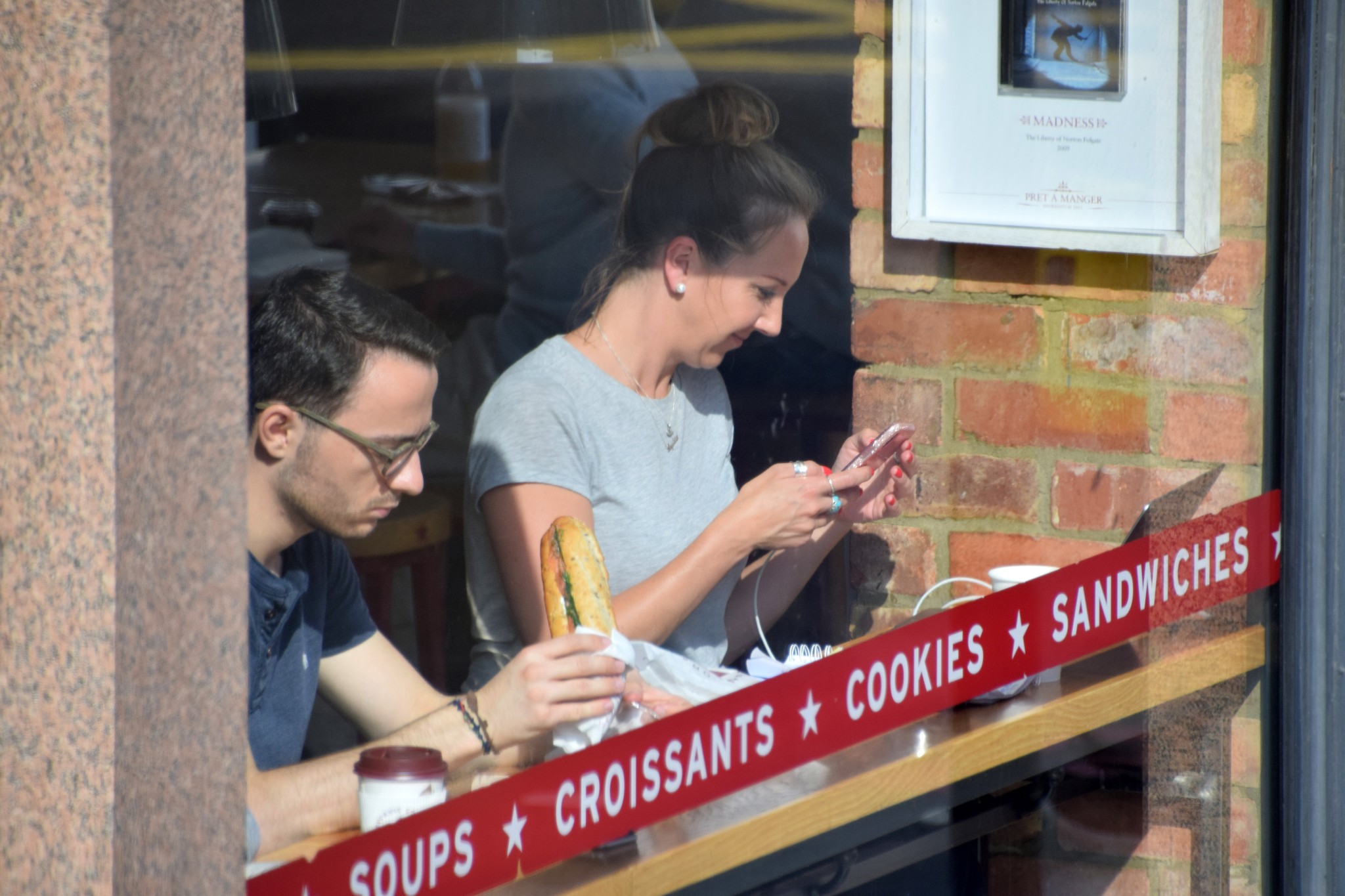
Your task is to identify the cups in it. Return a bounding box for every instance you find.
[987,564,1059,685]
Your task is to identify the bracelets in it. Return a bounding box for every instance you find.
[447,689,500,757]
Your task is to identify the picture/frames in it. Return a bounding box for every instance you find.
[891,0,1224,258]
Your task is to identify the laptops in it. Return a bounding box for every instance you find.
[1121,463,1226,545]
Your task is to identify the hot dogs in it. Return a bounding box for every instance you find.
[540,516,616,641]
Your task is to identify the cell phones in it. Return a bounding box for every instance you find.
[840,423,917,472]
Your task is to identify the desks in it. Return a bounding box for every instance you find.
[243,621,1269,896]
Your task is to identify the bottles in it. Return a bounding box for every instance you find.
[434,49,489,186]
[352,744,449,833]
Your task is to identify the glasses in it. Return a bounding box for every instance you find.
[257,400,440,478]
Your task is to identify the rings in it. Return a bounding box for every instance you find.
[892,451,902,464]
[790,459,808,478]
[826,493,841,517]
[823,473,836,496]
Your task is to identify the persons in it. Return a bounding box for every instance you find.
[460,75,916,708]
[245,265,696,878]
[470,0,705,368]
